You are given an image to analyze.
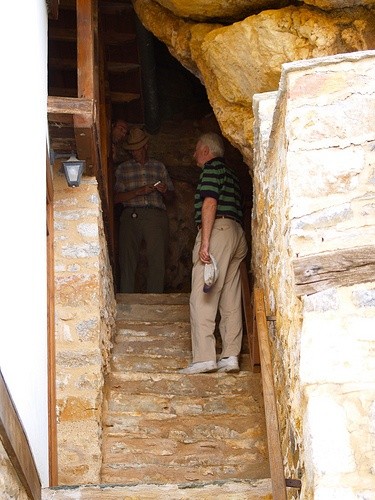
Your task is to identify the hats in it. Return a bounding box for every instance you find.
[122,127,150,151]
[202,253,219,292]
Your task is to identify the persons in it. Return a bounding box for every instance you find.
[111,118,128,163]
[178,132,249,374]
[111,126,171,294]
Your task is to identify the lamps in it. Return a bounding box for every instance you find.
[61,152,86,188]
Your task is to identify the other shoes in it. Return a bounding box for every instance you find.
[216,355,239,372]
[179,360,218,374]
